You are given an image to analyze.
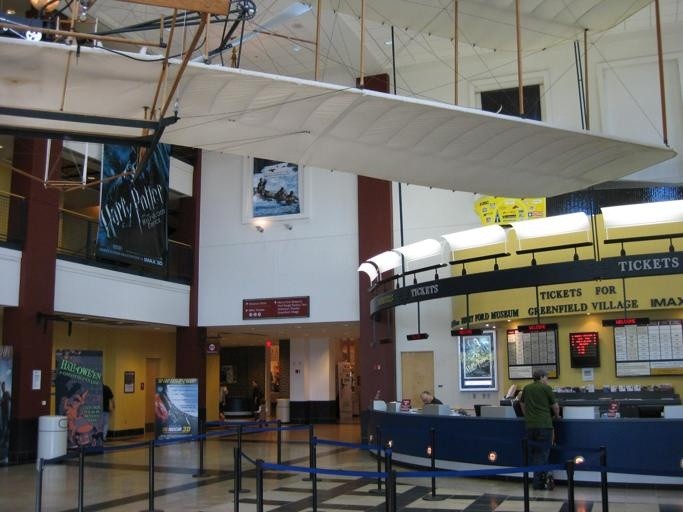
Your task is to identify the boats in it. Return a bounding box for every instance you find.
[256,188,295,202]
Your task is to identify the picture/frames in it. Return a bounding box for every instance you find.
[124,371,135,393]
[242,156,309,222]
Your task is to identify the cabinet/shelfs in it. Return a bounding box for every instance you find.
[337,362,360,424]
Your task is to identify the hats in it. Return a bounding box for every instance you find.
[533,369,549,378]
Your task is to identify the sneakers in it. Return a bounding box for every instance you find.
[545,472,554,491]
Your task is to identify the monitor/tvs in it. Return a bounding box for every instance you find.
[474,405,492,416]
[639,405,661,418]
[550,406,563,419]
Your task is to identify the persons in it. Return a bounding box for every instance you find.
[103,377,115,441]
[0,381,11,428]
[523,371,562,492]
[251,379,263,422]
[57,374,90,449]
[0,418,10,464]
[154,383,169,440]
[420,390,443,404]
[219,380,229,421]
[601,401,620,418]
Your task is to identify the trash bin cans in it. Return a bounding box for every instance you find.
[36,415,68,473]
[276,399,290,423]
